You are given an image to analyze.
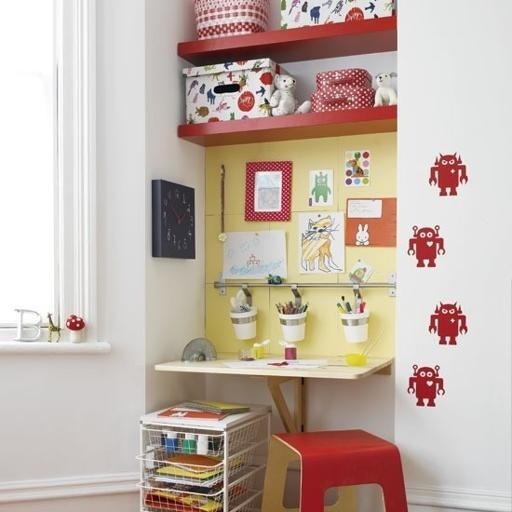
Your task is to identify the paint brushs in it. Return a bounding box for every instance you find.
[335,294,367,314]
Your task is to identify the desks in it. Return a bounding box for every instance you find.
[155,348,392,436]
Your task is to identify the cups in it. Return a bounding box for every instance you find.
[278,313,308,342]
[229,308,258,341]
[340,313,370,343]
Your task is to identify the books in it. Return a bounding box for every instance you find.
[154,459,246,484]
[156,396,251,425]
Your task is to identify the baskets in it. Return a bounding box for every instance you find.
[193,0,270,41]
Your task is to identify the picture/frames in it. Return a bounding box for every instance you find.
[245,160,291,220]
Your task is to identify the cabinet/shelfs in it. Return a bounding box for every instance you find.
[136,403,272,512]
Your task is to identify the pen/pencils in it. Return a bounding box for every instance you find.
[274,300,310,314]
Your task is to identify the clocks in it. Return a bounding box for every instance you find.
[151,179,196,260]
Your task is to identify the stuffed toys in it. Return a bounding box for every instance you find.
[372,71,398,108]
[269,73,297,116]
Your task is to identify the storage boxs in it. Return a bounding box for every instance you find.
[182,59,291,123]
[310,90,376,114]
[315,67,374,88]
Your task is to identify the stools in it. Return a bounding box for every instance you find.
[263,430,409,512]
